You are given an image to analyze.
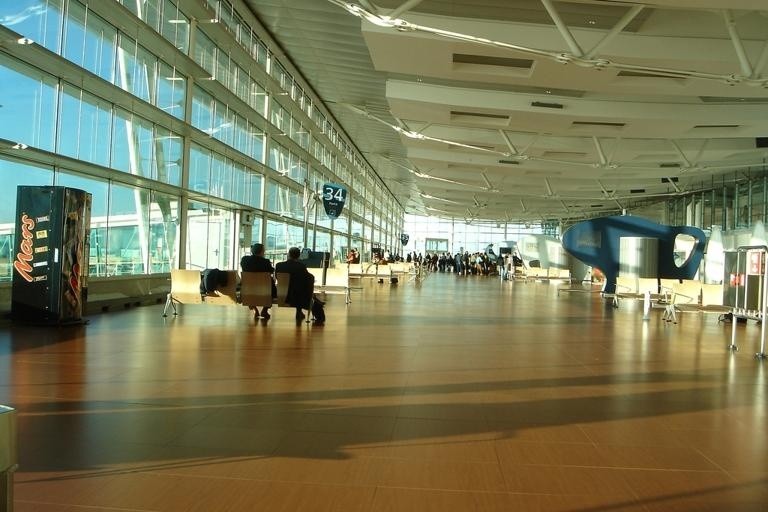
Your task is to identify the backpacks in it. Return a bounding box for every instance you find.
[313,302,325,324]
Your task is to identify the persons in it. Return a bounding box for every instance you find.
[240,244,326,322]
[378,249,400,283]
[407,251,510,279]
[348,248,360,264]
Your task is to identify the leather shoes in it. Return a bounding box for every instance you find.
[317,301,326,306]
[254,312,271,318]
[296,312,305,319]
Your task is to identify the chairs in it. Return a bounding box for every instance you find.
[306,267,352,304]
[387,261,414,276]
[603,277,723,324]
[163,269,318,322]
[515,266,571,285]
[335,264,392,284]
